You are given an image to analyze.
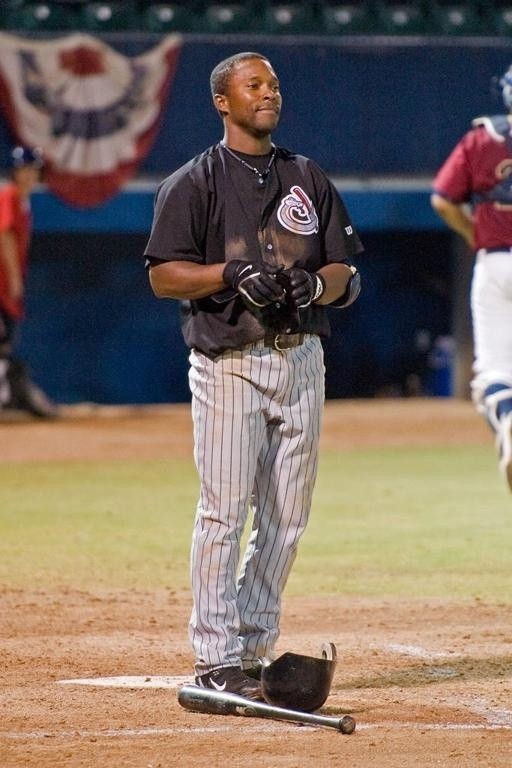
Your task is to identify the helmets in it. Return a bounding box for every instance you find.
[259,642,336,712]
[501,68,512,112]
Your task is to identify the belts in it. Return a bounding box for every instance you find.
[486,246,510,253]
[264,333,304,348]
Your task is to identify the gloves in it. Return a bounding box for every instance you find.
[223,260,284,306]
[276,268,326,307]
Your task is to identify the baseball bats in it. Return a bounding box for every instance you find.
[177,684,357,736]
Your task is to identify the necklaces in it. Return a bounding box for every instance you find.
[218,138,277,184]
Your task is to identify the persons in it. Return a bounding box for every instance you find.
[425,64,511,496]
[136,48,371,709]
[2,142,63,423]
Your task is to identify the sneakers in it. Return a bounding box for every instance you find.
[195,666,262,700]
[497,413,512,494]
